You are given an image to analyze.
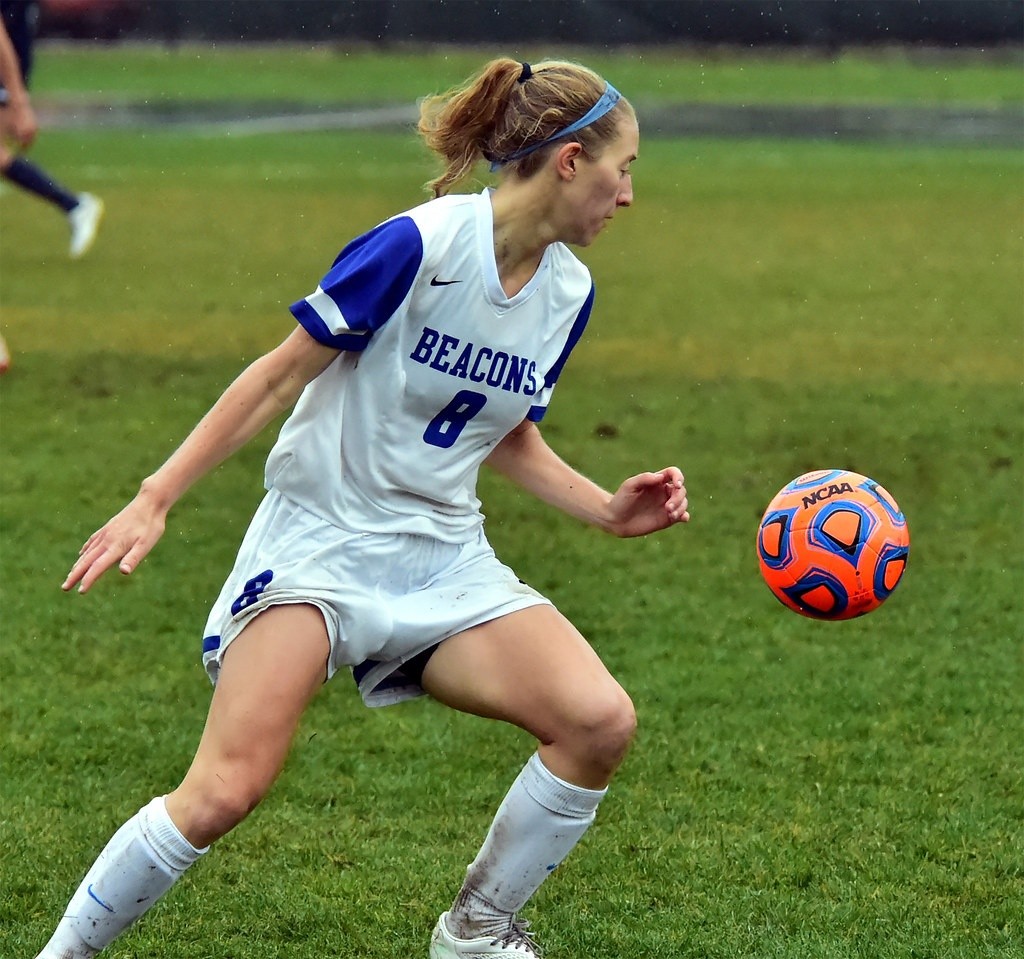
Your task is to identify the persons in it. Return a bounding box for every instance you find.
[0,0,105,370]
[34,57,690,959]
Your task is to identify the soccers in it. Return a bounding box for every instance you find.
[752,465,916,625]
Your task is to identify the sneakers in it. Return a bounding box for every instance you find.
[429,910,540,959]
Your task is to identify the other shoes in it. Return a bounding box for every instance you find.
[68,190,105,257]
